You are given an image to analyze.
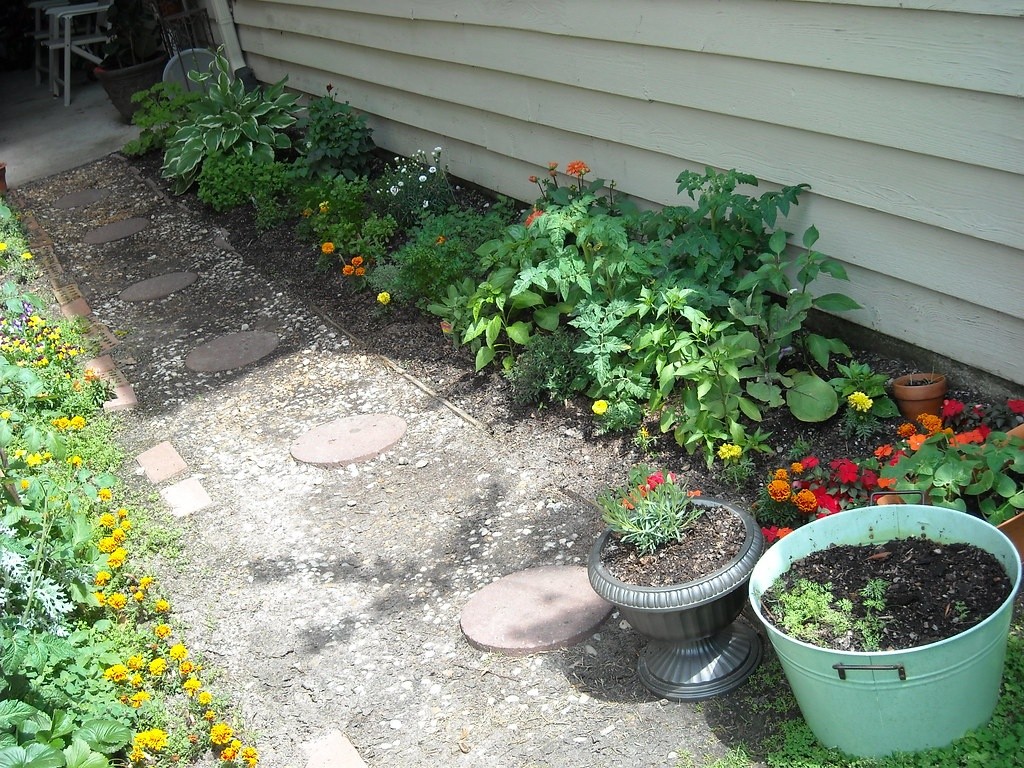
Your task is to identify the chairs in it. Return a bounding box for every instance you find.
[27,0,114,107]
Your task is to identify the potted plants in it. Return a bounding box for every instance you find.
[93,0,169,126]
[875,413,1024,561]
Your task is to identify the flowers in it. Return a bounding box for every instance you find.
[598,461,705,556]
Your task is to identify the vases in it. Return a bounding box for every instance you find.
[748,491,1023,758]
[0,162,8,194]
[893,373,946,419]
[588,497,762,701]
